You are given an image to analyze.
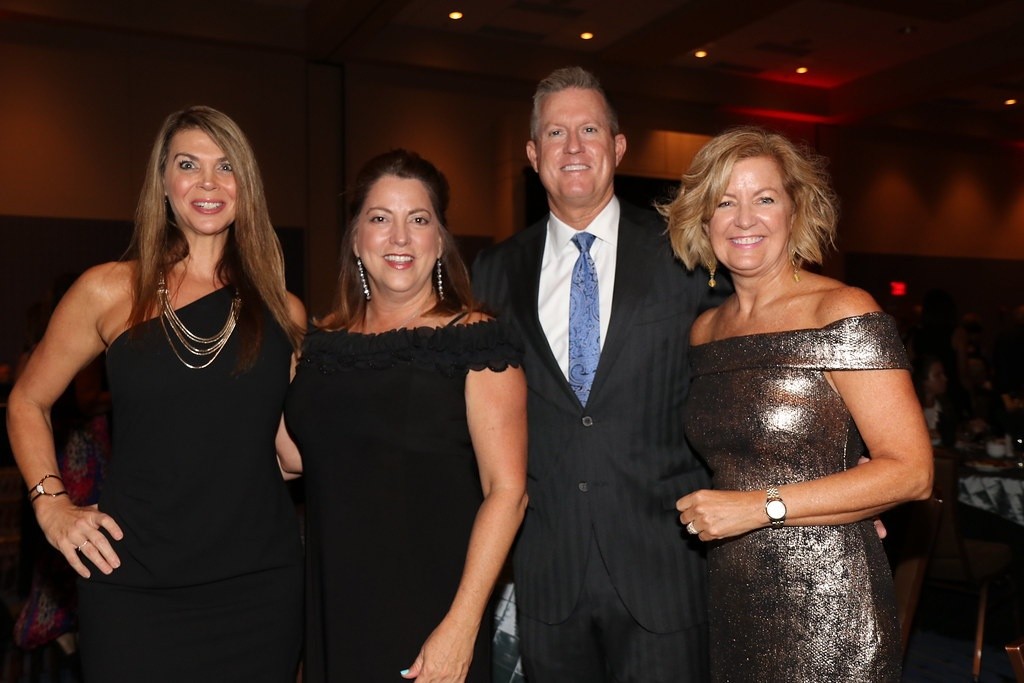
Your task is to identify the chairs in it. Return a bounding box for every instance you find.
[879,456,1024,683]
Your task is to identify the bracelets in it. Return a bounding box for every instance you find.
[29,474,69,504]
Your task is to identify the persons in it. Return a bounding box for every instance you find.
[653,130,934,683]
[872,285,1024,449]
[464,64,887,683]
[5,104,309,683]
[0,272,115,648]
[277,148,530,683]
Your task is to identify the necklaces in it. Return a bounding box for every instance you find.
[155,272,243,370]
[396,296,426,331]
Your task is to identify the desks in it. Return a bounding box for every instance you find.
[958,464,1023,533]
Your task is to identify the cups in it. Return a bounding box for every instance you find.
[986,443,1005,459]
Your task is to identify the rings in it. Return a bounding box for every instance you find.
[687,522,699,535]
[78,540,88,551]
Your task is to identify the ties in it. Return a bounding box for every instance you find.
[568,233,600,407]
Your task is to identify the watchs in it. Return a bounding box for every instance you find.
[765,485,788,529]
